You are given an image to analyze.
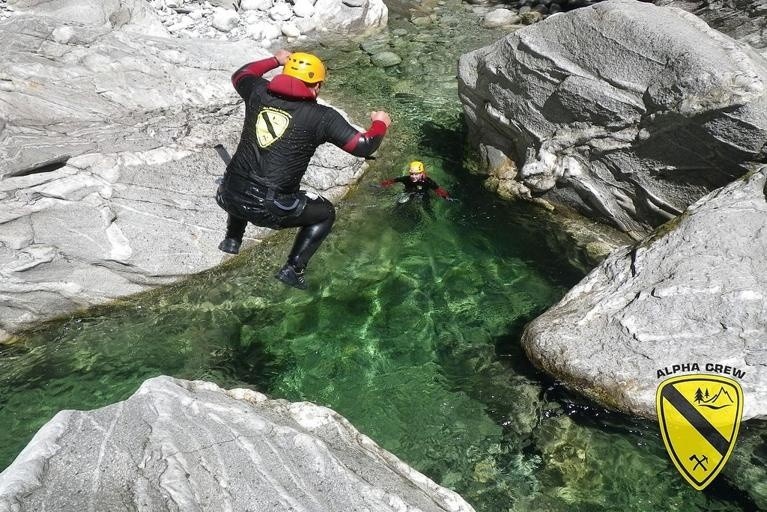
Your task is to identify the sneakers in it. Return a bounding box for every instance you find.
[275,264,307,289]
[218,239,241,254]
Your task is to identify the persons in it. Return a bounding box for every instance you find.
[379,161,458,220]
[214,50,391,290]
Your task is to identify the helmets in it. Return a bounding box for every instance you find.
[282,52,325,84]
[409,161,424,173]
